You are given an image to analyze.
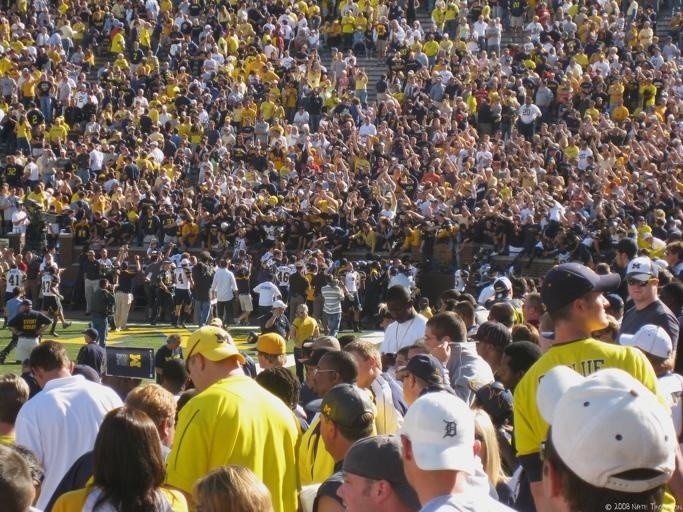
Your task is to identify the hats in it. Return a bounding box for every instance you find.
[0,150,682,498]
